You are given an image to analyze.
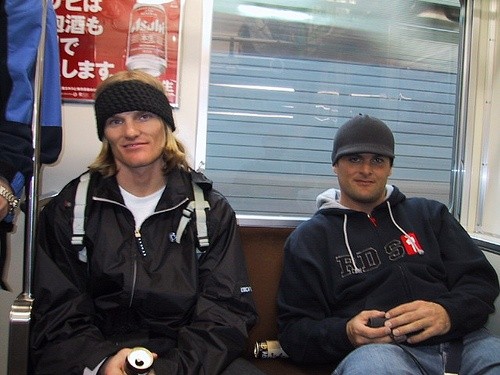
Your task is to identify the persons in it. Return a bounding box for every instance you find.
[276,116,499,375]
[29,70,261,374]
[0,0,64,293]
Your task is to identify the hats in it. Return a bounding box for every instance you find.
[332,112,395,165]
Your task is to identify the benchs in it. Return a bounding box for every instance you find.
[234,227,334,375]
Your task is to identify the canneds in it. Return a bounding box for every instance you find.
[254,340,290,359]
[125,1,168,77]
[121,347,154,375]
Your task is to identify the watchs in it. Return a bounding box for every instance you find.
[0,186,20,210]
